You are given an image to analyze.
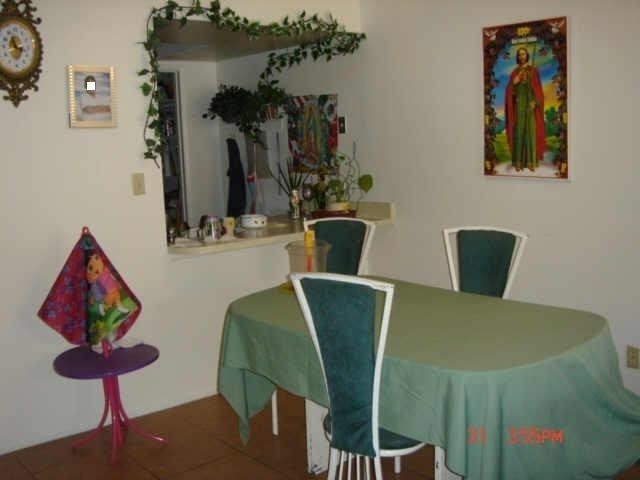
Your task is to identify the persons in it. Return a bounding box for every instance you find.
[79,76,111,120]
[503,46,546,173]
[82,248,130,319]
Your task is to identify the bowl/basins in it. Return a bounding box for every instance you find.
[240,214,267,228]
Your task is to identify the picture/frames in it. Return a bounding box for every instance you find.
[67,64,120,129]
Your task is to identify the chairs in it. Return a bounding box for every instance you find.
[442,226,528,300]
[302,217,377,276]
[288,271,427,480]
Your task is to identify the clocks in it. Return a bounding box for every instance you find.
[0,0,44,107]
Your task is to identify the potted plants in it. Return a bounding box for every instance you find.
[312,145,374,218]
[255,80,292,119]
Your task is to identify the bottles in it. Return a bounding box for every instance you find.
[206,216,220,243]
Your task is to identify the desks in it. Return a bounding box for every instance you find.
[218,275,640,480]
[53,344,170,470]
